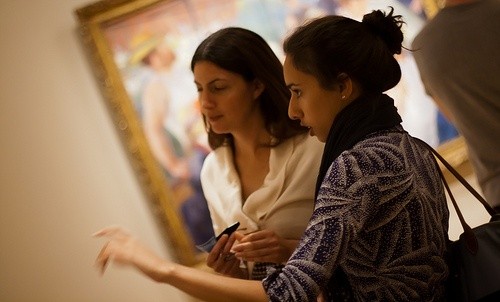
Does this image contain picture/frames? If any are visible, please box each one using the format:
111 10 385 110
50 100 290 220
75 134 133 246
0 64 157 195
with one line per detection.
77 0 469 266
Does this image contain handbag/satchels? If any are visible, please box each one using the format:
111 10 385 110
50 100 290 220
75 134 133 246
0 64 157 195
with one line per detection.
412 136 500 302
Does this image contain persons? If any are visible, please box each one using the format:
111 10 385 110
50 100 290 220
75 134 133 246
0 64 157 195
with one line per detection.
93 7 450 302
412 0 500 213
191 27 325 280
